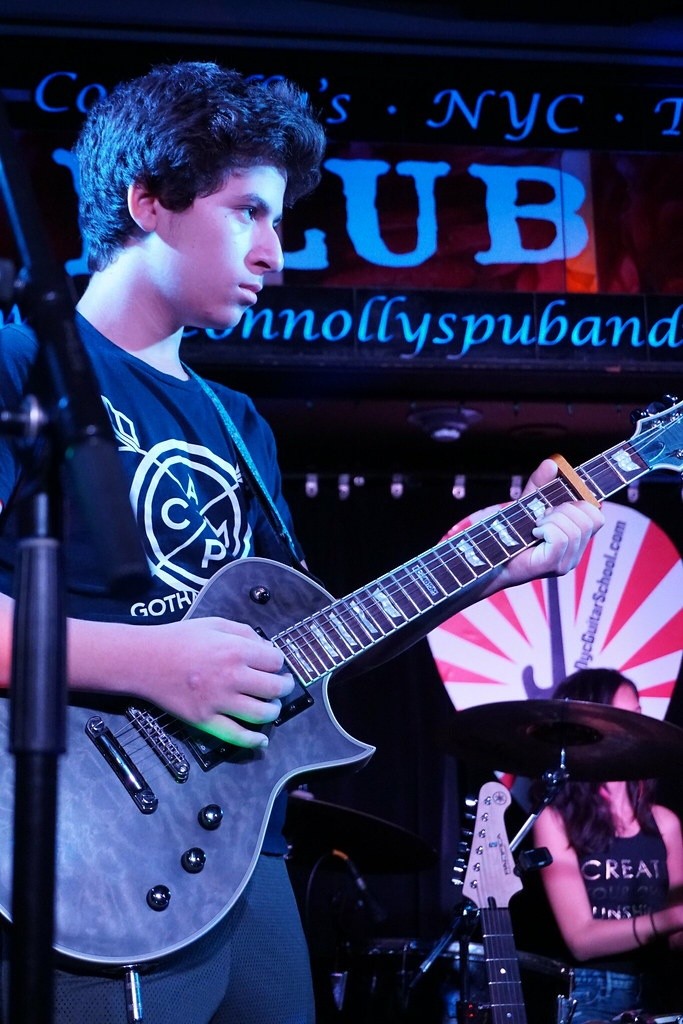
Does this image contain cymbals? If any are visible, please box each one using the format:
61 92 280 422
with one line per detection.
453 694 683 784
286 790 411 844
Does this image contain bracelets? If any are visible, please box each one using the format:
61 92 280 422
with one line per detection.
633 911 659 948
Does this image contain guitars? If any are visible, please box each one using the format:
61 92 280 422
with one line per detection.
0 388 682 983
449 780 532 1023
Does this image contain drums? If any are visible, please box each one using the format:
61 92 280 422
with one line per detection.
363 933 578 1024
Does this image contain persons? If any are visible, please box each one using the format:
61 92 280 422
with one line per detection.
534 669 683 1024
0 62 603 1024
